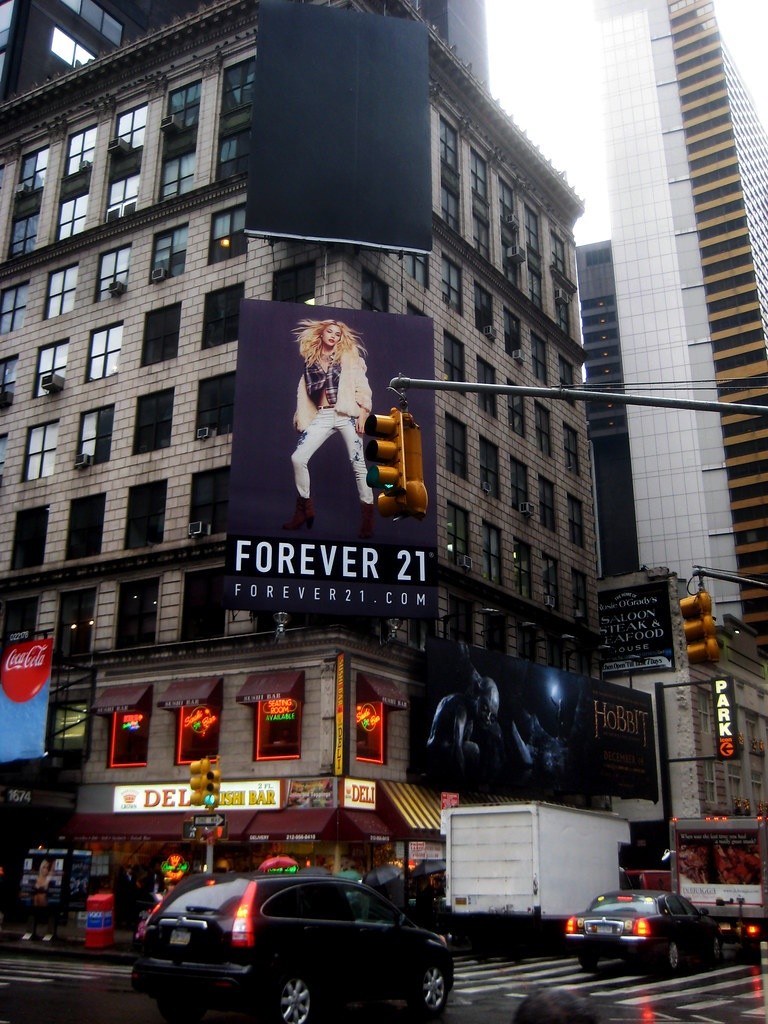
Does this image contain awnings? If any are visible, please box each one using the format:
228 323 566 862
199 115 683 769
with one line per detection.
236 671 305 710
357 674 409 713
157 678 223 717
90 684 153 720
56 810 397 846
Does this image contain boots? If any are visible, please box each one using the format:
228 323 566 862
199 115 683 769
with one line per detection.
284 496 315 529
360 502 375 538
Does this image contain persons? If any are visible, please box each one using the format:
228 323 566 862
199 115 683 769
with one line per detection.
33 859 54 906
283 319 375 538
118 865 138 930
510 989 602 1024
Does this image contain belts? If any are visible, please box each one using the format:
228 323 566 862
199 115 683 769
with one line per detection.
318 405 335 409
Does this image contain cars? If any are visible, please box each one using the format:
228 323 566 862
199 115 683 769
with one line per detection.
132 873 455 1024
564 888 724 972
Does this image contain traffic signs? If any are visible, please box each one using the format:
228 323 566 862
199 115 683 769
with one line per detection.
193 813 227 827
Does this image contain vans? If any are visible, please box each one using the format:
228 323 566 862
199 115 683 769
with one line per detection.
626 869 672 892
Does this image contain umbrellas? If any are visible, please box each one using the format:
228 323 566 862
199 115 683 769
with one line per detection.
297 867 332 875
259 855 296 871
366 864 401 887
334 870 361 880
412 860 446 876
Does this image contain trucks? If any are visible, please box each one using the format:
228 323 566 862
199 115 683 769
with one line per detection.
668 816 767 939
439 800 633 956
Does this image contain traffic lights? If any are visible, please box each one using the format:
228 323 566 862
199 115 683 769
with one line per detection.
679 591 720 664
204 769 220 808
189 759 210 805
362 407 405 519
